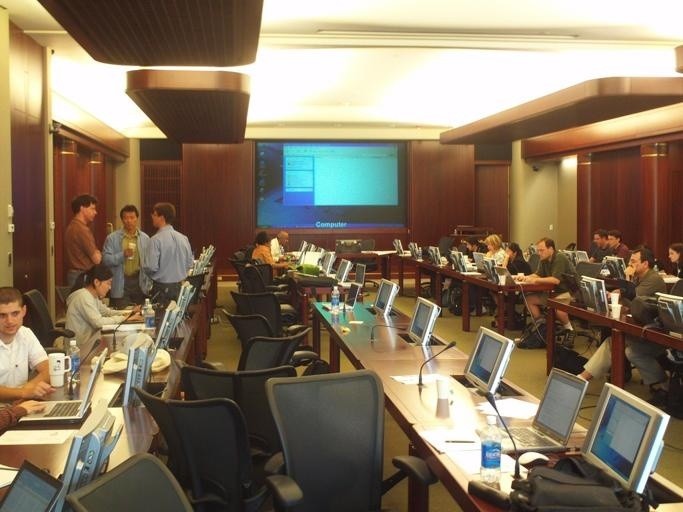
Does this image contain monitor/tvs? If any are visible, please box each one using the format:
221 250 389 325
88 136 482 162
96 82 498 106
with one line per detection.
462 325 517 395
581 380 671 494
407 295 441 345
577 250 589 264
55 397 124 512
296 239 366 287
579 275 609 313
605 255 626 279
371 279 401 317
393 236 519 286
175 279 197 318
123 332 158 407
193 242 216 276
653 290 683 331
154 300 182 351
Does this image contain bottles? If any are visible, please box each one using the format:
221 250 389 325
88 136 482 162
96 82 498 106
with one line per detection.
480 416 502 490
331 286 340 322
145 304 156 339
653 258 659 272
141 298 150 313
66 340 80 382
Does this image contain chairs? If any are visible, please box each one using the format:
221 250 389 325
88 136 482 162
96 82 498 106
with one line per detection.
234 251 245 258
670 279 683 297
183 359 327 475
254 262 274 283
264 370 439 512
230 290 306 336
135 386 285 512
575 262 605 278
64 452 193 511
528 254 539 273
57 285 75 309
436 235 456 254
201 327 312 377
25 289 74 349
228 257 250 290
222 309 318 368
234 263 297 320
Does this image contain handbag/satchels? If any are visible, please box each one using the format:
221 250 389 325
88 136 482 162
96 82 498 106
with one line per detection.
507 453 644 512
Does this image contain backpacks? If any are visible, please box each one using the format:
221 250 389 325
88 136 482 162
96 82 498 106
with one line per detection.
516 316 547 349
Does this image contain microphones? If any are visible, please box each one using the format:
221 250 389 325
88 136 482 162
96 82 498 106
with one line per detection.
112 307 137 354
69 337 100 396
342 293 370 315
483 387 525 483
368 322 408 342
419 336 459 397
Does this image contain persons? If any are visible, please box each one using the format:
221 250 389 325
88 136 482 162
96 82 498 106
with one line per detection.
450 227 683 420
104 205 152 311
146 202 196 335
1 399 45 432
271 231 295 263
0 287 56 401
252 231 289 279
244 240 258 259
60 194 103 291
66 263 141 364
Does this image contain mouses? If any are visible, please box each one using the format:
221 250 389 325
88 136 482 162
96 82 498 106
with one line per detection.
520 442 553 466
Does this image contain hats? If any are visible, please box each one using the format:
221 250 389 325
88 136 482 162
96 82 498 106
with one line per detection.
100 332 171 376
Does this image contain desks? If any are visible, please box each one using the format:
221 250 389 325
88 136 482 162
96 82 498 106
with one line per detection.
286 250 391 282
281 259 367 345
393 250 555 334
1 310 199 497
311 301 683 512
187 259 219 357
605 267 679 296
545 295 682 397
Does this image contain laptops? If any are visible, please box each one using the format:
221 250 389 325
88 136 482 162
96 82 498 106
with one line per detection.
18 344 110 427
0 458 64 512
499 365 589 453
322 282 360 312
561 271 584 304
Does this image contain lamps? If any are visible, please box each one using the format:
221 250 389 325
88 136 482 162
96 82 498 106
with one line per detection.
88 151 104 164
59 139 78 155
640 144 668 158
576 154 601 166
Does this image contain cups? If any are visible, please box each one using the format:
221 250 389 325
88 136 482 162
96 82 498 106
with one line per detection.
612 306 620 320
48 353 71 387
128 243 136 259
518 273 524 282
611 293 620 304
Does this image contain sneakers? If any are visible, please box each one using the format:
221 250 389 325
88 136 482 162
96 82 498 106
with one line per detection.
647 379 670 408
561 328 577 348
